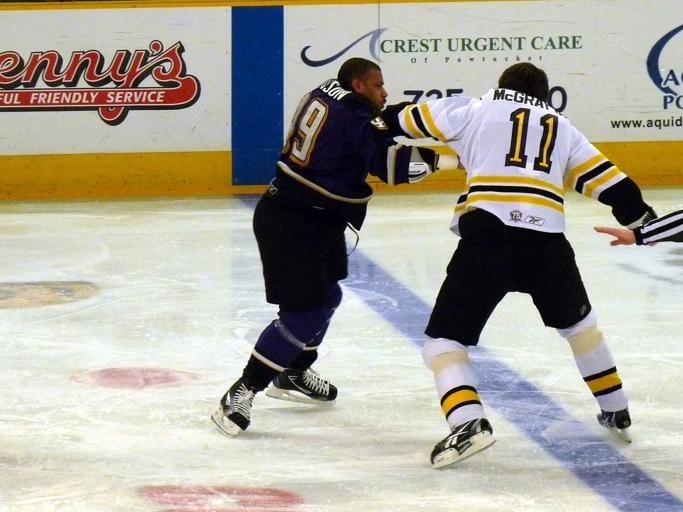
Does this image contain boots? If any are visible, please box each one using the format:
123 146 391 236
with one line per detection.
210 376 258 438
265 367 338 406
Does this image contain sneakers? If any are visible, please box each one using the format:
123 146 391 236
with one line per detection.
596 408 633 443
431 417 496 470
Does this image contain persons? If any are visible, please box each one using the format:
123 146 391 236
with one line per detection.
204 58 463 430
591 207 682 246
384 60 654 465
